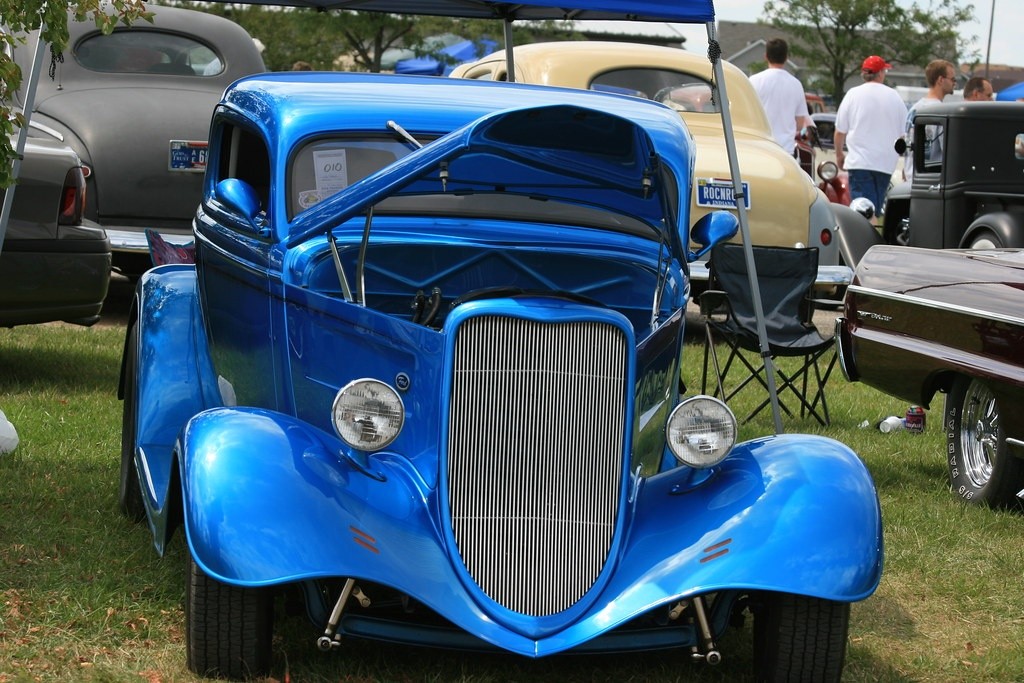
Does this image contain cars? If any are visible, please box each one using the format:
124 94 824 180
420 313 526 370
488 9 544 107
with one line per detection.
0 128 114 330
448 39 884 289
0 0 270 280
396 32 500 77
660 80 1024 252
833 246 1024 514
115 69 883 683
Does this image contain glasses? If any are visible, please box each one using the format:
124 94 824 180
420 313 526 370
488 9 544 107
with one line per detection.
883 68 888 73
943 76 956 82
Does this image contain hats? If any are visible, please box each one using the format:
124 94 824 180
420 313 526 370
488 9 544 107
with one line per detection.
863 56 891 73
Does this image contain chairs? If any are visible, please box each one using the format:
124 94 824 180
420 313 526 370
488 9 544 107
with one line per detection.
694 241 846 427
150 62 198 77
293 146 397 216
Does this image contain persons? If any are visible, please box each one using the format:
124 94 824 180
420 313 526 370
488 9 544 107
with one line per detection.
749 38 809 160
834 56 908 225
963 77 996 102
904 61 964 188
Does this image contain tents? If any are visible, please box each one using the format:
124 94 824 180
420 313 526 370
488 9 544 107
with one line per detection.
997 81 1024 100
395 38 500 76
0 0 785 439
